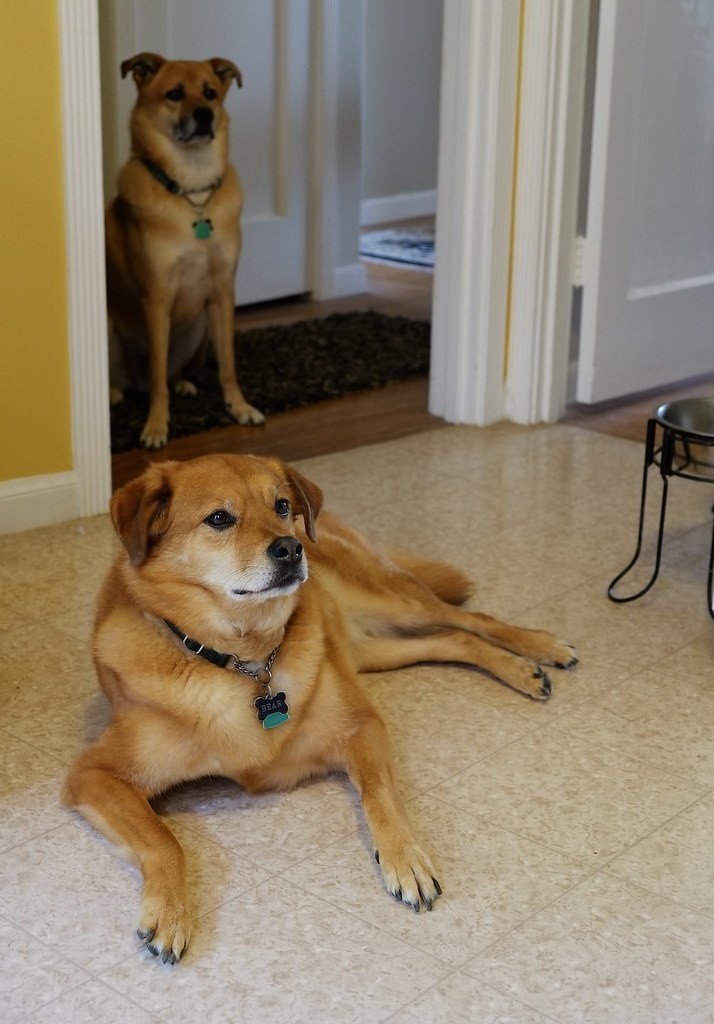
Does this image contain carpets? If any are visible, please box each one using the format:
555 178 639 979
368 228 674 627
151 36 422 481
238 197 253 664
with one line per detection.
111 309 430 451
359 221 435 272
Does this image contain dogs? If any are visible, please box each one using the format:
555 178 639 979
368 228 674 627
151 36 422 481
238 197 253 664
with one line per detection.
61 452 581 966
104 50 266 450
